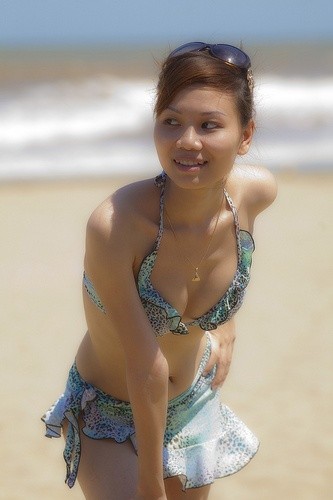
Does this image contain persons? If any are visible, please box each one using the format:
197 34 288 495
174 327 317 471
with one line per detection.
41 42 278 500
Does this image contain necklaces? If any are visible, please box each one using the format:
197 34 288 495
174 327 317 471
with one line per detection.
164 192 225 282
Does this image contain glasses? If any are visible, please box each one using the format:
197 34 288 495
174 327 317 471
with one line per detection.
168 41 252 72
